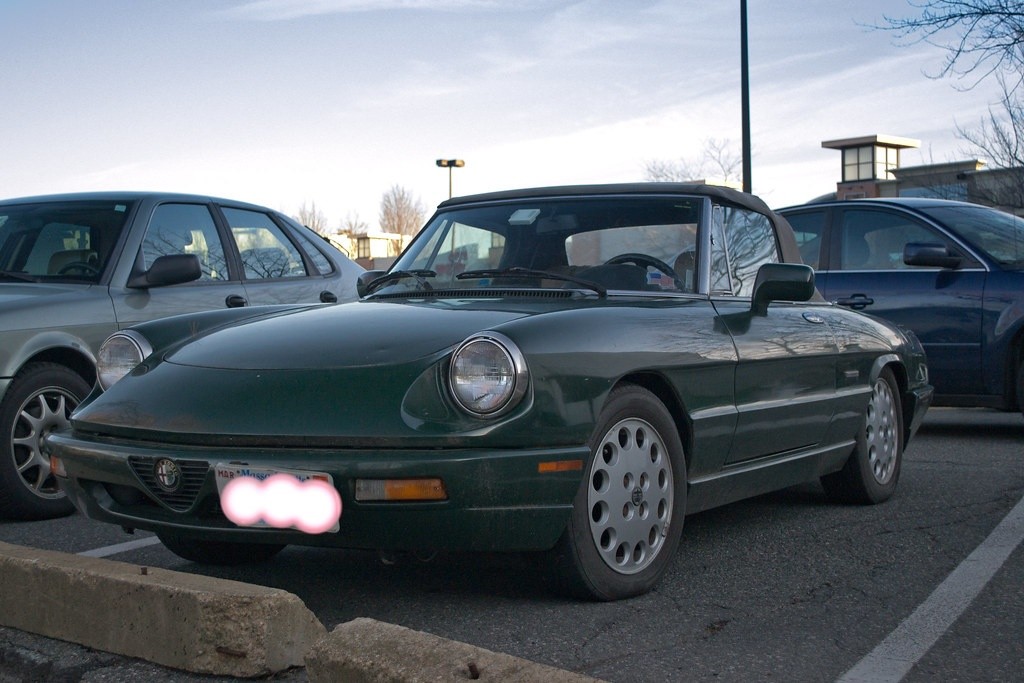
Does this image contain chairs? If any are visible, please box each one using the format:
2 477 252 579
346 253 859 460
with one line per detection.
674 251 696 293
841 237 873 270
48 250 97 277
560 265 648 290
240 248 290 278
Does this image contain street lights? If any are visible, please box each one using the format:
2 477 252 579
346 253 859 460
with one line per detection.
437 159 466 256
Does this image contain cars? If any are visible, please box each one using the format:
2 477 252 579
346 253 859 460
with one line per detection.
651 198 1024 411
0 193 367 519
37 181 932 600
